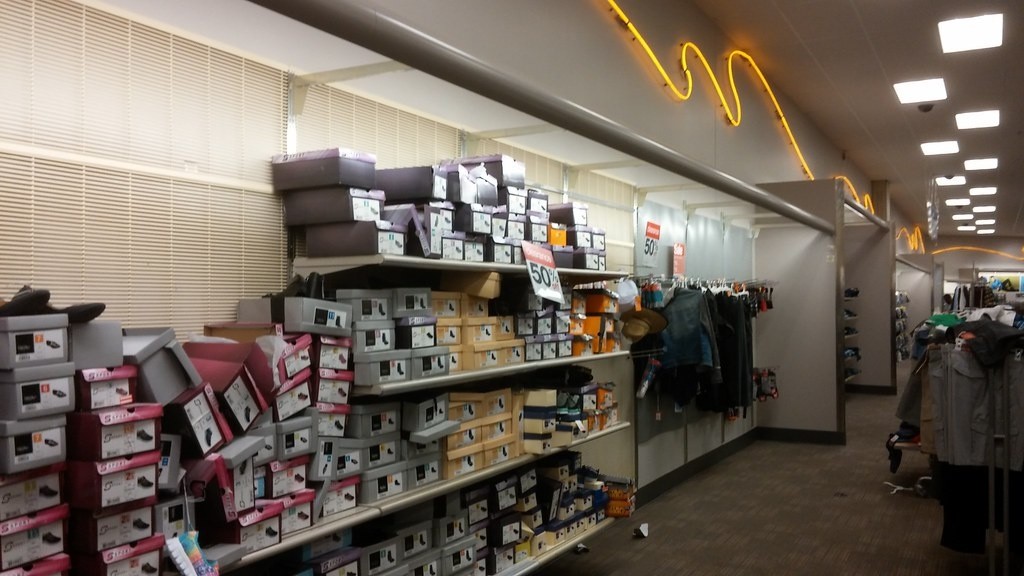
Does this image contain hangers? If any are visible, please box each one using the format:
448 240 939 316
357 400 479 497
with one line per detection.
629 274 750 299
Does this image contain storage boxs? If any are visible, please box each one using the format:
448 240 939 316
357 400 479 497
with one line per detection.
0 147 633 576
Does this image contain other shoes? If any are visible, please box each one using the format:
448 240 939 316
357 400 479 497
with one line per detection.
306 273 323 299
0 285 50 316
264 277 307 318
45 298 105 323
165 530 219 576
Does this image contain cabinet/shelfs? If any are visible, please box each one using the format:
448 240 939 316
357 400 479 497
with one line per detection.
842 294 861 382
216 254 633 575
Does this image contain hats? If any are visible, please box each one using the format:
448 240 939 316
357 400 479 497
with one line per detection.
618 308 667 342
614 277 639 320
